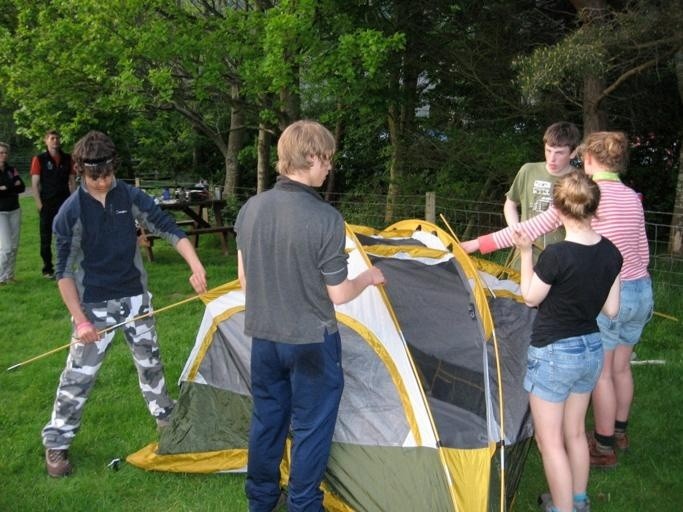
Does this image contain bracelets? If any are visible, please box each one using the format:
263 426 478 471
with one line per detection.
75 321 94 332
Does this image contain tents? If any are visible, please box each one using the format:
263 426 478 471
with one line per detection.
6 219 535 507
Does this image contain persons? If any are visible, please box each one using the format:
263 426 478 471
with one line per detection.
0 141 26 286
458 131 652 468
31 131 73 277
41 132 208 478
504 123 583 274
233 120 384 508
513 170 624 508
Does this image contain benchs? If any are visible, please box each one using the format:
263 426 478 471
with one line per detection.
135 225 241 247
131 218 202 236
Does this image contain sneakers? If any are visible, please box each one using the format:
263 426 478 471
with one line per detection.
42 271 56 279
537 490 591 512
44 446 72 478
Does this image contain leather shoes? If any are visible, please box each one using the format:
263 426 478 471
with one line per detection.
587 433 630 453
588 447 618 469
